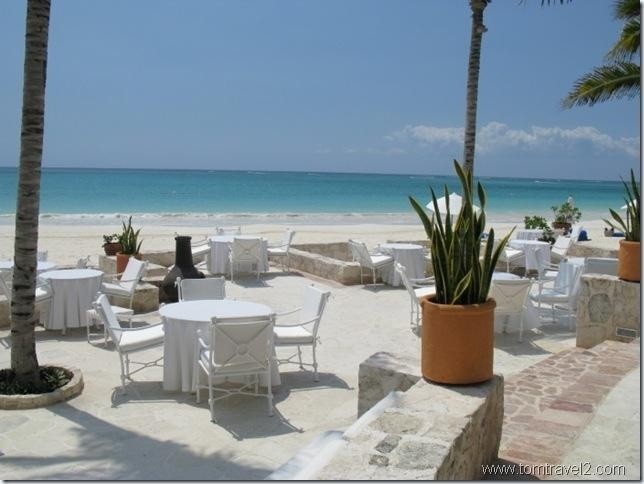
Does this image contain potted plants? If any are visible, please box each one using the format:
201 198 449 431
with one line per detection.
102 234 121 255
409 160 517 385
117 217 144 273
601 168 641 283
550 203 581 235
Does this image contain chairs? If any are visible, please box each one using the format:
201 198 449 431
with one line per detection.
0 251 164 395
157 227 331 424
348 223 585 343
